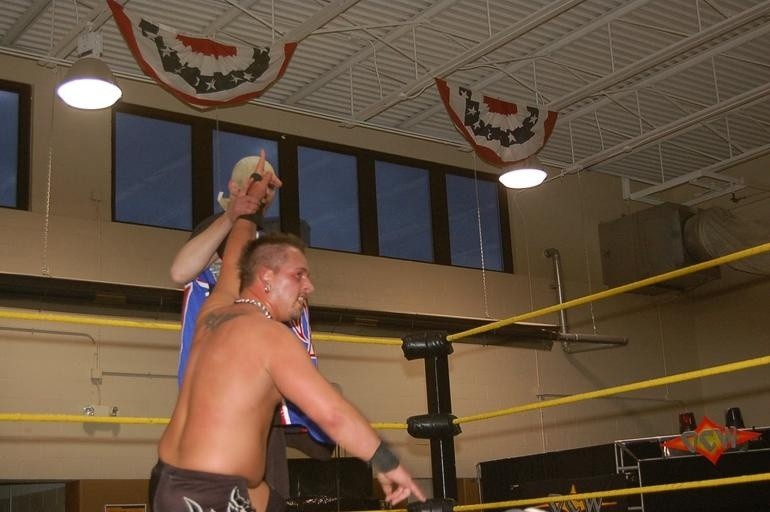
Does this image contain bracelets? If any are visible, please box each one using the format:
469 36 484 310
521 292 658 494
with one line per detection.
370 444 401 473
239 196 264 229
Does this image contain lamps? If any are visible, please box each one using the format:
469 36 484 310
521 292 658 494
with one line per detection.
55 18 123 111
499 152 547 189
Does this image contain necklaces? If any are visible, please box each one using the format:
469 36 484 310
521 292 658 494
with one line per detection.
233 296 274 321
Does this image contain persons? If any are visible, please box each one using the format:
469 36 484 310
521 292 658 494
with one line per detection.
168 156 332 512
147 148 428 511
